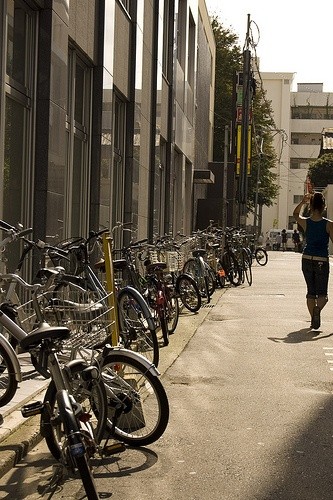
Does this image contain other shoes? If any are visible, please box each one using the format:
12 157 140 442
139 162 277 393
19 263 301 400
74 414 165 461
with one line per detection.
295 251 298 252
312 306 320 329
283 250 284 252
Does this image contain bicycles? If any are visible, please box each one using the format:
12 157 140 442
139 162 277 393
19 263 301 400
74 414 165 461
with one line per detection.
0 266 126 500
0 219 268 447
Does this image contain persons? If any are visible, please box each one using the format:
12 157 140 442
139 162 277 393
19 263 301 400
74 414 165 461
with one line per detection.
275 232 281 251
293 192 333 329
292 230 300 252
281 229 288 251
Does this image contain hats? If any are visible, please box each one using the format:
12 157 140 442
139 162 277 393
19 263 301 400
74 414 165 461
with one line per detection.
294 230 296 232
282 229 285 232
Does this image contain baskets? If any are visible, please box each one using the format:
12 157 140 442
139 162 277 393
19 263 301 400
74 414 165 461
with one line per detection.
149 251 184 272
48 236 88 276
17 290 115 349
180 236 196 248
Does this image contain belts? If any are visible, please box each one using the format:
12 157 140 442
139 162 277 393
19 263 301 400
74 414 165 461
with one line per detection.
302 255 327 261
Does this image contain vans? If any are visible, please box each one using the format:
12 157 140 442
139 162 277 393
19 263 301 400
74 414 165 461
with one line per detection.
269 229 303 251
